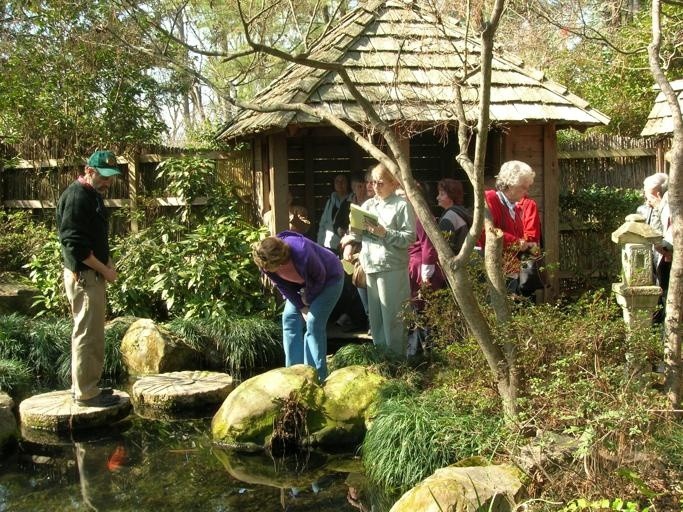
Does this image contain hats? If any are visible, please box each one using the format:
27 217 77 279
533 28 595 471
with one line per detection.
86 150 124 178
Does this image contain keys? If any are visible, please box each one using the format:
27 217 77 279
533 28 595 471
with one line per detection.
77 271 86 288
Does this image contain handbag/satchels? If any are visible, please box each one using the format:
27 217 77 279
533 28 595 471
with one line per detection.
350 257 367 289
519 251 553 298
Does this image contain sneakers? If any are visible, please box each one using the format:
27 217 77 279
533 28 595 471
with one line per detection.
334 313 353 328
72 387 114 404
75 392 121 407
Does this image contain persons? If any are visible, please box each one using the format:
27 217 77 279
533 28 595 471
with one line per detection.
56 151 123 406
634 173 674 330
